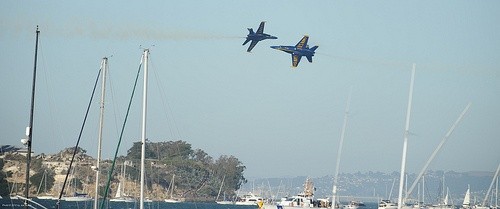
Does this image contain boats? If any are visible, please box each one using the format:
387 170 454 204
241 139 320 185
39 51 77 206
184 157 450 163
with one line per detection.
212 64 500 209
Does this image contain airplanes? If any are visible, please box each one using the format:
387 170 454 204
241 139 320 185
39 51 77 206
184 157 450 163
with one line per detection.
242 20 279 53
269 33 319 68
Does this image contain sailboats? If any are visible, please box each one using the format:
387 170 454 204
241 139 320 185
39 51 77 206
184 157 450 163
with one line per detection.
164 172 182 204
